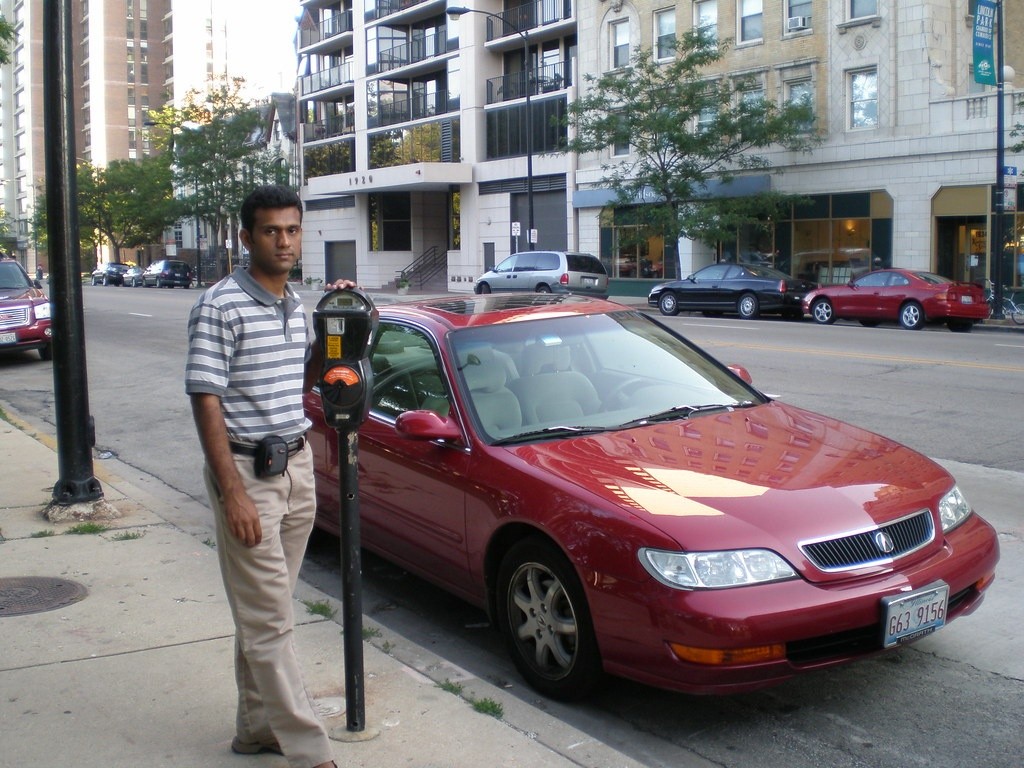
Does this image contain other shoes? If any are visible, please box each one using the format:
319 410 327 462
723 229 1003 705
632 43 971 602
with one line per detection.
231 736 283 754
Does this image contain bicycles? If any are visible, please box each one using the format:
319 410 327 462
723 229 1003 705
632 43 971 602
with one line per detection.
984 278 1024 325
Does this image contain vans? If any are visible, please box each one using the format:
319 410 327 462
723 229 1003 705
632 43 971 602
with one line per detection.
474 250 610 301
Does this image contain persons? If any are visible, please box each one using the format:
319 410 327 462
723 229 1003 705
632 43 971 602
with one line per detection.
186 183 357 768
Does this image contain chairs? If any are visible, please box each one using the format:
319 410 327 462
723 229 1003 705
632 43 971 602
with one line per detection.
421 346 521 431
507 341 606 425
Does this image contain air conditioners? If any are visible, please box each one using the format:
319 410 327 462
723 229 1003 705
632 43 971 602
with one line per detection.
787 15 808 30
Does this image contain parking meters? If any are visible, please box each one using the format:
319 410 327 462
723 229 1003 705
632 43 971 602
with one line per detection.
313 286 381 731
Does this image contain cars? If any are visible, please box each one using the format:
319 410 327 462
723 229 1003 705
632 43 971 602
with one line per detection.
121 266 146 288
802 268 991 333
91 263 131 286
0 259 53 361
142 260 192 289
303 293 999 705
647 263 817 322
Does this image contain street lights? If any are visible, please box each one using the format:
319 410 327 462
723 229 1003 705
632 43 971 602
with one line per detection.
446 7 535 251
144 122 202 288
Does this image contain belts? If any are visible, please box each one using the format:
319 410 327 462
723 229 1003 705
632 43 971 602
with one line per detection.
229 432 307 455
